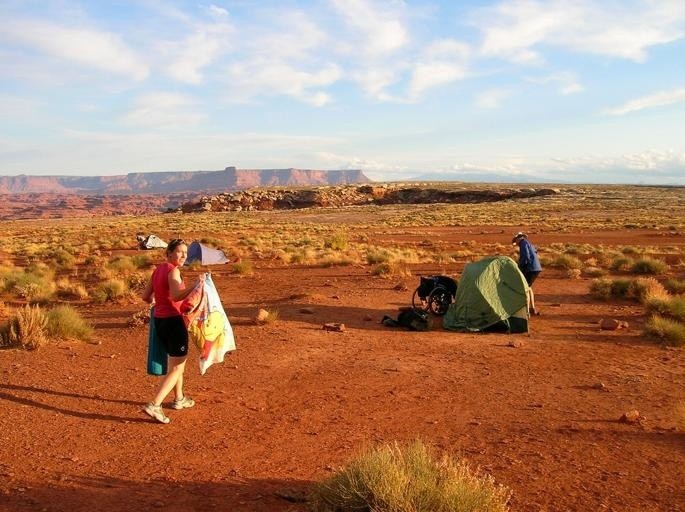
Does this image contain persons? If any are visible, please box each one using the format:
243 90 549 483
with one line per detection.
512 232 542 320
142 239 206 424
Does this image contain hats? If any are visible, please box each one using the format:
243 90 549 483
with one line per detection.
512 232 528 244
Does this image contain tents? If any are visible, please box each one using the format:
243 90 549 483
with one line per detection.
185 242 230 266
142 234 168 249
443 256 530 332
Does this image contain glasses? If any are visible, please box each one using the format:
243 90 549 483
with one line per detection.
169 238 186 246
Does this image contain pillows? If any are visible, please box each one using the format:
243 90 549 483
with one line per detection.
173 273 238 376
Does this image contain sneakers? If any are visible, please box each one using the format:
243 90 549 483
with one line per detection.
171 395 195 410
143 401 169 423
529 307 536 314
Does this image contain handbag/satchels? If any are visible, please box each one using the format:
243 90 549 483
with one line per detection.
384 307 433 331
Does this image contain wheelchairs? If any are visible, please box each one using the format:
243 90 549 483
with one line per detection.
412 271 452 315
